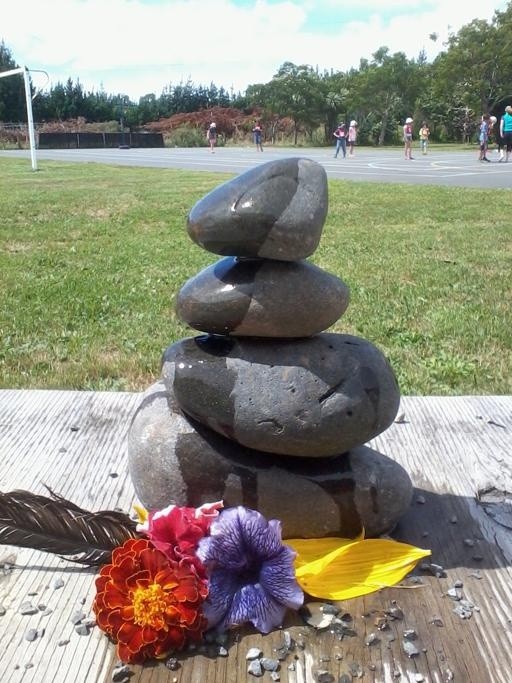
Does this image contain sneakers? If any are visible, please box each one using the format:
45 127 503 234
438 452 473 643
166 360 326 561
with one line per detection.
479 154 505 163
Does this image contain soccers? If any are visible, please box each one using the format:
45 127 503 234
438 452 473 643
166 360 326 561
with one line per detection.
490 116 497 124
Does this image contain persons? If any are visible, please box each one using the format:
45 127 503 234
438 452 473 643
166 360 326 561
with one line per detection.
483 114 497 163
251 119 264 151
418 121 430 155
332 121 349 159
479 113 488 160
348 119 358 156
402 116 414 159
497 105 511 162
206 120 218 152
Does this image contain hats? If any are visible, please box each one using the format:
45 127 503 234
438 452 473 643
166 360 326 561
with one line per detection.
337 120 358 128
404 118 414 123
421 120 428 128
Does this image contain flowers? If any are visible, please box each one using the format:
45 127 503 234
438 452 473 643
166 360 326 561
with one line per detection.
91 498 432 663
